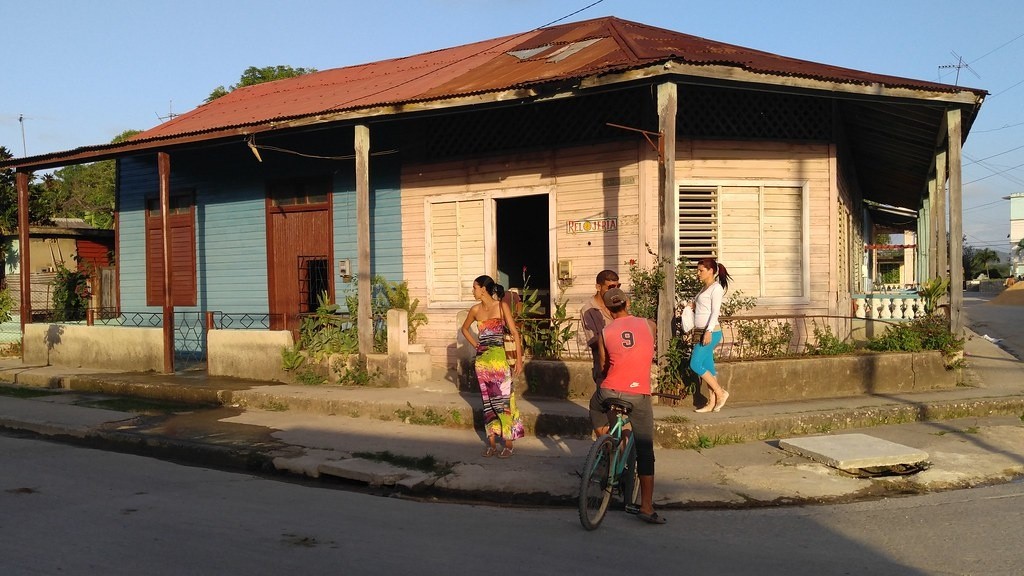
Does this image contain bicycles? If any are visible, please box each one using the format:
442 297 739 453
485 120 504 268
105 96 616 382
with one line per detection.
577 392 682 531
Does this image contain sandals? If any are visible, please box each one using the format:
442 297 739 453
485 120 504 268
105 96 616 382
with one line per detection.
482 447 499 458
498 448 516 458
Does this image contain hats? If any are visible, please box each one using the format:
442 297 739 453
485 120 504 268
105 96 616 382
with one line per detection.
603 289 627 307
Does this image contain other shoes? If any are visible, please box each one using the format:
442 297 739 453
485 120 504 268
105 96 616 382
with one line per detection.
694 391 730 414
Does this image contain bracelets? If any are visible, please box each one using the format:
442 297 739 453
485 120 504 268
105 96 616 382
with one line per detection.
706 330 712 333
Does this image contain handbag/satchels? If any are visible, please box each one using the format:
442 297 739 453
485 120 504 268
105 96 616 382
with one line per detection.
682 305 696 333
503 334 525 368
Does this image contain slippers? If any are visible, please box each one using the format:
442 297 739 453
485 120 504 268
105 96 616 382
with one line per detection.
637 512 667 524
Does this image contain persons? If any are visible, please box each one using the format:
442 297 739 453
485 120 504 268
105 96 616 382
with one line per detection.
686 258 729 413
581 269 631 366
461 275 525 458
589 288 667 524
1006 275 1014 287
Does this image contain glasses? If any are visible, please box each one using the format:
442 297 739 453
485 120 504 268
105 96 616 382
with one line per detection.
603 282 622 290
